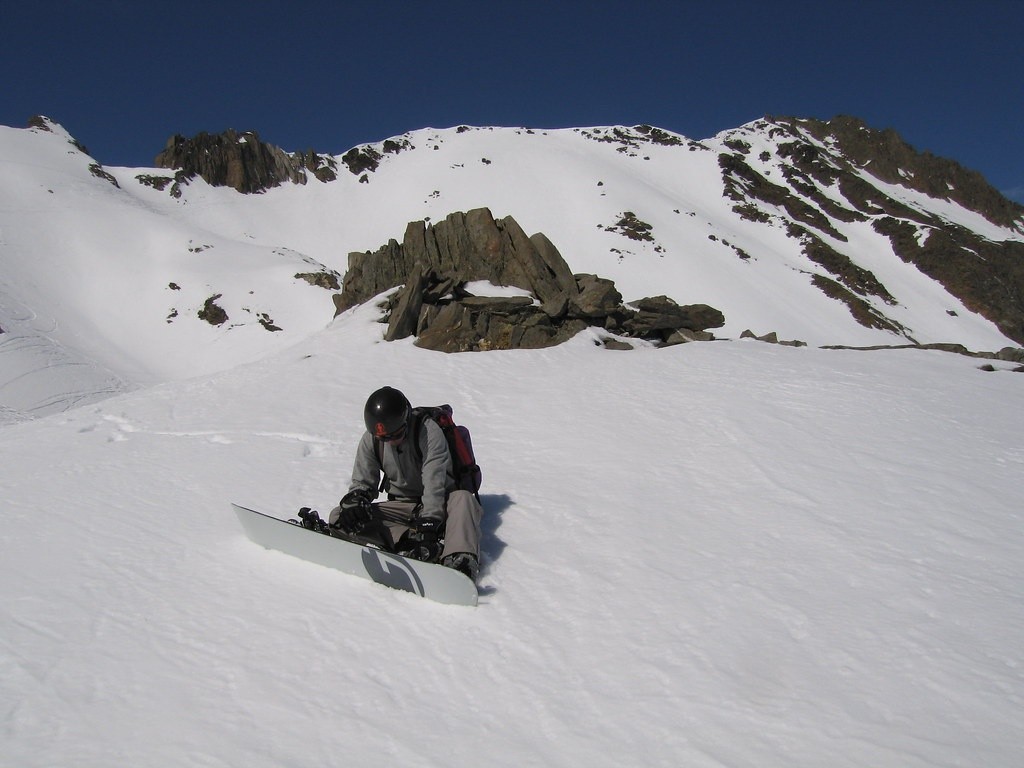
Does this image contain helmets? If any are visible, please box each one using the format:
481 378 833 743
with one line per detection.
363 386 412 437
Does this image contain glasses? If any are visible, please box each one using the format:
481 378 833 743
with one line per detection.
376 426 409 442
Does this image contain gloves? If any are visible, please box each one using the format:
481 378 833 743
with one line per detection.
339 489 373 537
408 517 445 562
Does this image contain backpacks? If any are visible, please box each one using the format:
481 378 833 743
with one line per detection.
411 404 482 506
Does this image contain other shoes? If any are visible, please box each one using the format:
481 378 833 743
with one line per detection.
437 552 480 584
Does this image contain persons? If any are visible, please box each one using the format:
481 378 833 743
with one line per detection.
328 386 483 580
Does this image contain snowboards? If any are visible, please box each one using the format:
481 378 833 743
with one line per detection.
229 502 479 608
